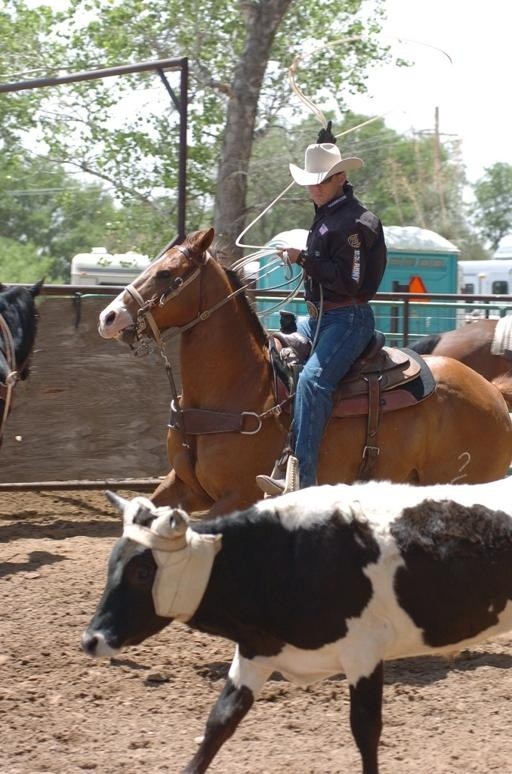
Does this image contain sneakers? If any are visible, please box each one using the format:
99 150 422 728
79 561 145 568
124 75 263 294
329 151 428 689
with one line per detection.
256 474 318 497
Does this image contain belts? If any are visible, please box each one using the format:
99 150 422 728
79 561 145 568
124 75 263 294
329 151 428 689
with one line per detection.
308 293 368 313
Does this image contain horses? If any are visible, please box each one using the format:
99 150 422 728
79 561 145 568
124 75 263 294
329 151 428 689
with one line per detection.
0 274 47 448
404 315 512 378
91 222 512 516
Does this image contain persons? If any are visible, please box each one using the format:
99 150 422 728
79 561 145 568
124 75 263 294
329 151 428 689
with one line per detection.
256 143 388 497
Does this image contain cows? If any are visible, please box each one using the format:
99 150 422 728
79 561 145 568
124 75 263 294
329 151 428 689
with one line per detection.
78 466 512 774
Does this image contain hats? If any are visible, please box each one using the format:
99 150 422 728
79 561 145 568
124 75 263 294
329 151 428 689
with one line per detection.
288 143 365 186
